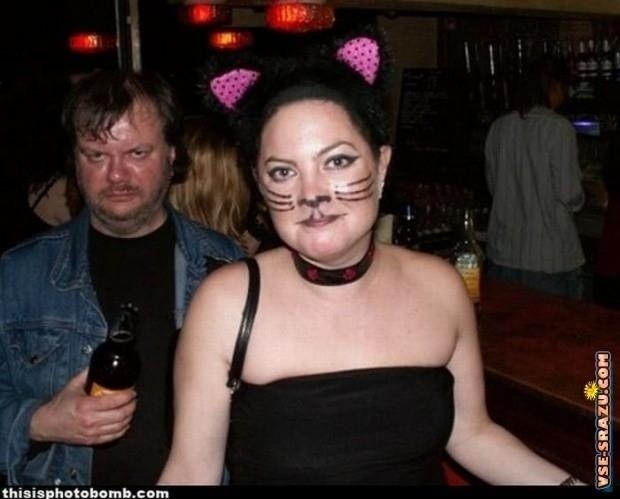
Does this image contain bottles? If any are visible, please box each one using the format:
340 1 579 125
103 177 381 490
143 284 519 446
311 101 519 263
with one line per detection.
399 204 420 252
540 34 620 99
81 301 145 401
453 206 486 329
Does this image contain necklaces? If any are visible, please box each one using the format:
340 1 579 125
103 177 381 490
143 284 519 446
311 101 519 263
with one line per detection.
288 231 376 285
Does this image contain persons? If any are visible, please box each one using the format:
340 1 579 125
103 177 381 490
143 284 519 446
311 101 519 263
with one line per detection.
0 144 84 253
238 176 282 256
479 54 598 302
156 37 594 487
592 134 618 309
1 64 247 487
169 111 253 241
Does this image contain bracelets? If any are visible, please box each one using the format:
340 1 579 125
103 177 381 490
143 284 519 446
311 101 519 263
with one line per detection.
559 472 580 485
